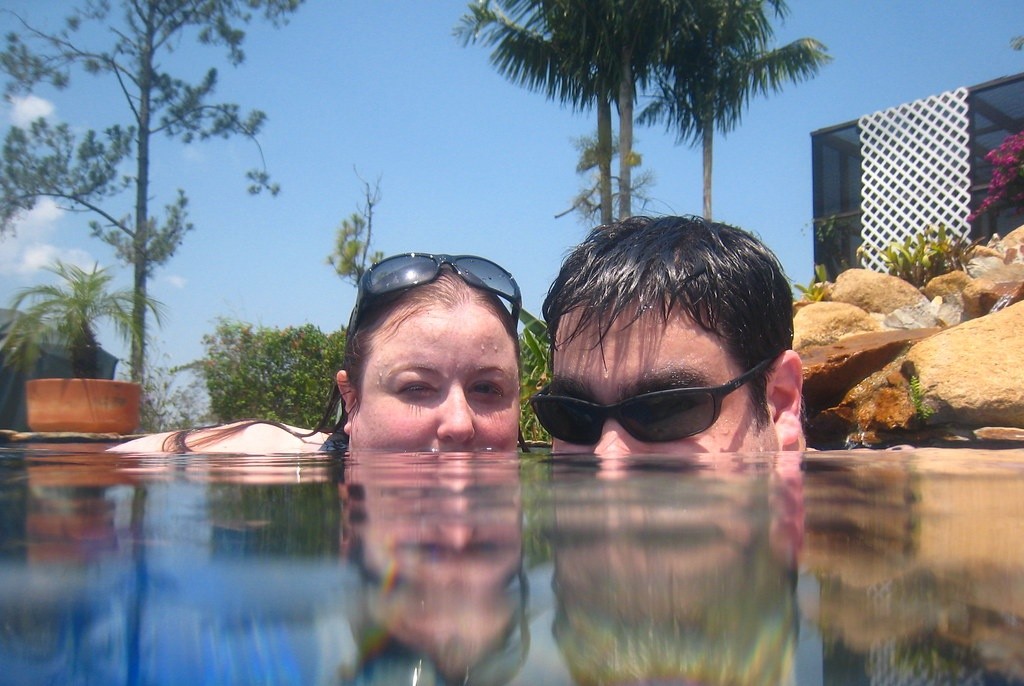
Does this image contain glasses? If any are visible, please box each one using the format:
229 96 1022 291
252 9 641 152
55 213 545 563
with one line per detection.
529 356 777 445
346 254 523 345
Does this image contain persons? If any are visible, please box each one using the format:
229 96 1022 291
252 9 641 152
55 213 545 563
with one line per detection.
531 216 818 454
103 252 520 453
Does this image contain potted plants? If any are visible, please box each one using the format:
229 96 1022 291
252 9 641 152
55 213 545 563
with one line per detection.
8 464 173 683
0 257 168 431
